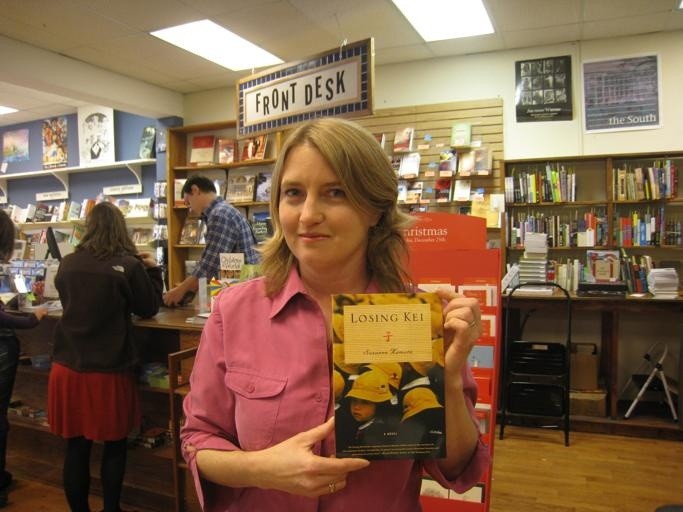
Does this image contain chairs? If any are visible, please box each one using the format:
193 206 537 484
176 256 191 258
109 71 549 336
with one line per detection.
615 340 679 423
498 282 573 446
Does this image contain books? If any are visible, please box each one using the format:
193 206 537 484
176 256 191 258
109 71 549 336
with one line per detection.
503 164 576 204
371 133 385 157
18 349 45 367
437 147 492 177
245 211 275 245
621 245 650 294
612 205 682 245
8 398 47 427
645 266 678 297
393 129 414 153
330 292 447 456
613 158 678 201
132 420 167 450
500 232 581 294
397 177 483 205
189 136 216 162
134 358 169 390
173 175 275 204
472 195 504 228
388 154 420 178
217 137 238 163
450 124 471 147
505 208 607 249
242 133 267 161
5 200 153 221
177 212 208 245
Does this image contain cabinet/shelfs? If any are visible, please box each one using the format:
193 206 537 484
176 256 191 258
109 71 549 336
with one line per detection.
0 158 167 305
504 153 683 437
167 117 290 305
0 305 215 512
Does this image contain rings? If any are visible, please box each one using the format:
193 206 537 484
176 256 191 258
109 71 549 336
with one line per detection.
329 483 335 493
469 322 477 327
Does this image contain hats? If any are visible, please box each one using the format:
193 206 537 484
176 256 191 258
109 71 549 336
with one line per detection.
361 362 403 390
430 339 446 369
409 352 435 378
333 344 361 374
333 293 444 343
344 372 394 402
400 387 444 422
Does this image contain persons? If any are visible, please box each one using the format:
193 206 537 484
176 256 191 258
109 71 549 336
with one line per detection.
179 117 489 512
161 176 256 306
47 202 163 512
0 208 48 507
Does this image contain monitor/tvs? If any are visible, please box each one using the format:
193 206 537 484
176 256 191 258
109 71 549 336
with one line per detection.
45 227 62 261
